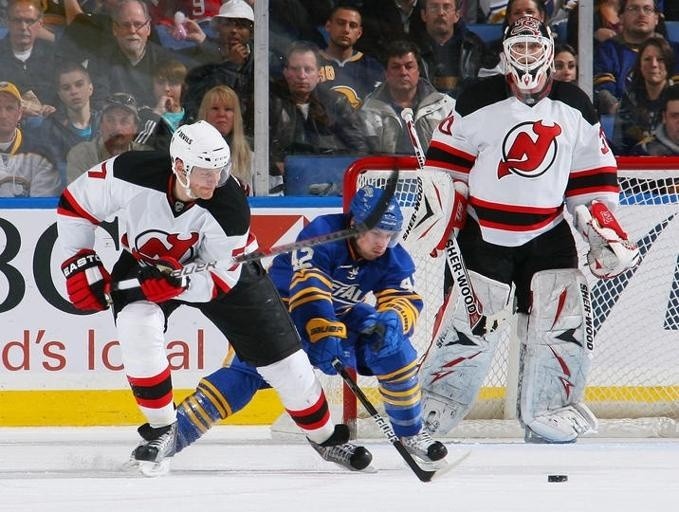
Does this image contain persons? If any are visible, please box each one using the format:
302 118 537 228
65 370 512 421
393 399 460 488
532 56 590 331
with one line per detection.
135 184 448 463
0 0 679 198
401 16 640 444
56 120 372 471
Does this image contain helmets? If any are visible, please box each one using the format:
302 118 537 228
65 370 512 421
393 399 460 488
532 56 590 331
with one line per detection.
502 14 554 91
350 184 403 231
169 119 230 189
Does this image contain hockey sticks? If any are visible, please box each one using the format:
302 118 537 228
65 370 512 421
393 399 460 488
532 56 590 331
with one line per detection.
401 106 526 336
103 167 400 298
332 360 483 484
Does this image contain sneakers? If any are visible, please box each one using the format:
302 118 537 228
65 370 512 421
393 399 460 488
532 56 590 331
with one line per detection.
135 423 178 462
401 432 448 462
307 425 372 470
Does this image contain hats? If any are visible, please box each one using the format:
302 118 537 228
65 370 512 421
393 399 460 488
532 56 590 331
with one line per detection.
213 0 254 21
0 81 21 100
103 91 139 117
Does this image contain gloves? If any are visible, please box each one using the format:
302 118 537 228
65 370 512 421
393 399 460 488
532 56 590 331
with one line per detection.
61 249 111 311
360 310 401 360
310 337 347 375
138 256 189 303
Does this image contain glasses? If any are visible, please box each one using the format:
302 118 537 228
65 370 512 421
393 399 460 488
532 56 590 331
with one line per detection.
104 95 135 105
